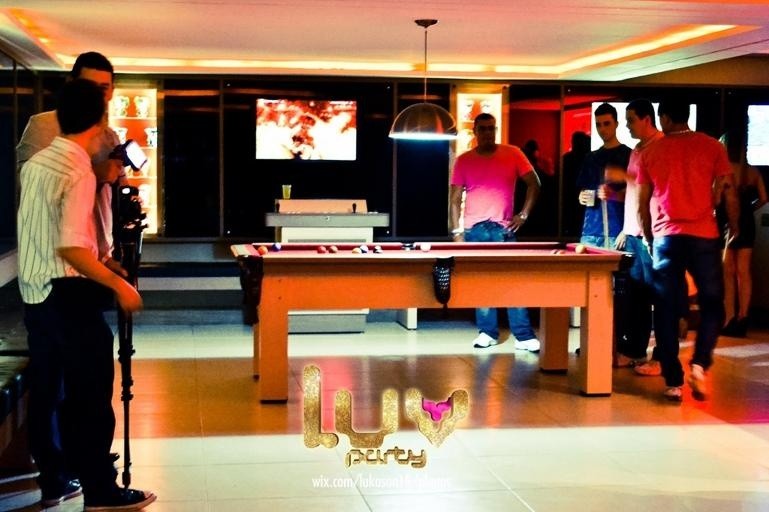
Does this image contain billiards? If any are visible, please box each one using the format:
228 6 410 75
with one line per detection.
352 245 368 253
329 246 337 253
373 246 381 253
317 246 326 253
272 243 281 251
257 246 268 255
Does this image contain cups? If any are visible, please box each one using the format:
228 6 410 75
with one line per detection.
585 190 596 207
282 184 292 199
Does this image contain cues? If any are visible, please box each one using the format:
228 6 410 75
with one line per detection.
601 199 609 247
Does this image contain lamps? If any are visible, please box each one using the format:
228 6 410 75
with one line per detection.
384 17 463 141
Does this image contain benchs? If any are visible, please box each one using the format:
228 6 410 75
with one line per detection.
274 197 370 215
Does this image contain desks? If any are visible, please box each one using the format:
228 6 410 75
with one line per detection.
225 236 632 406
265 209 392 244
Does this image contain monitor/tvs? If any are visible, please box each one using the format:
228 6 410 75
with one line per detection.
253 95 359 165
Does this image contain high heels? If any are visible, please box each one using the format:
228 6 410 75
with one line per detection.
721 315 748 337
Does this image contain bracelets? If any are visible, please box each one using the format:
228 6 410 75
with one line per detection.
450 227 461 238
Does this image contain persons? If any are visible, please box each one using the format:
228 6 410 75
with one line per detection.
288 136 312 160
16 78 158 511
14 52 123 472
450 113 544 352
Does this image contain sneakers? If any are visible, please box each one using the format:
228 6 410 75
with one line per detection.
473 331 499 347
636 360 664 375
664 385 682 401
108 450 119 463
613 351 648 368
514 337 541 353
85 480 156 511
41 480 84 506
